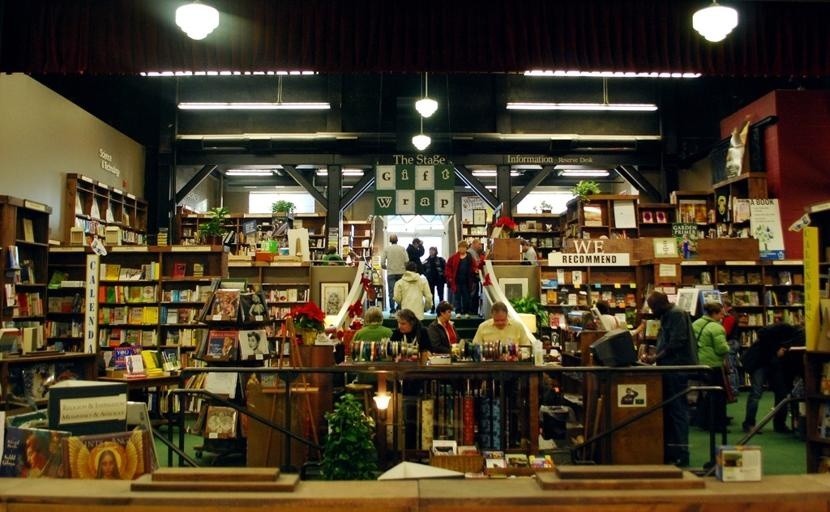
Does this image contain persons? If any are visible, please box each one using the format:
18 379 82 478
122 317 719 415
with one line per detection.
722 309 751 404
640 289 699 468
350 234 541 449
691 302 734 437
738 321 805 434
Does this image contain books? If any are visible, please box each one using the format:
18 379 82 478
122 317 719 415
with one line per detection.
532 461 706 492
541 268 803 388
553 460 685 479
130 467 302 492
151 466 280 483
1 178 371 478
463 199 785 263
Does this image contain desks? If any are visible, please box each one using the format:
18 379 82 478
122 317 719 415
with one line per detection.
238 362 711 474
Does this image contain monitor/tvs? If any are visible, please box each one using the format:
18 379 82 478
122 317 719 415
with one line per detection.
588 329 639 367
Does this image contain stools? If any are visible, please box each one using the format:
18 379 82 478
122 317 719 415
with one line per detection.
344 382 374 418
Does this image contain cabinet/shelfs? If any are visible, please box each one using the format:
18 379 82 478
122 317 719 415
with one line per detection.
475 87 830 474
1 167 384 434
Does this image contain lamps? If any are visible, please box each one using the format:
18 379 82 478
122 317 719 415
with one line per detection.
411 115 432 151
523 0 706 81
372 391 393 410
140 0 360 144
691 0 739 43
415 71 438 118
